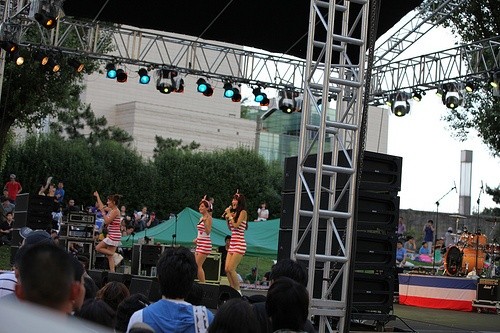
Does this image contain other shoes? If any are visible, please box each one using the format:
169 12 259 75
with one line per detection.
237 290 242 297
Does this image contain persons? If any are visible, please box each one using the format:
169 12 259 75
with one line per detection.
94 191 124 272
257 200 269 221
0 174 81 245
0 230 315 333
93 199 159 263
193 199 213 284
218 235 231 277
222 193 248 297
245 265 276 287
253 217 258 222
396 217 486 268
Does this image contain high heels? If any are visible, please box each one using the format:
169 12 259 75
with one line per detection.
112 252 124 265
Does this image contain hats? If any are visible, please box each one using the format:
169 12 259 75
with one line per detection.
10 174 16 179
19 227 54 243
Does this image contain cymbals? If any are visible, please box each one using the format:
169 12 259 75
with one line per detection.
449 215 467 219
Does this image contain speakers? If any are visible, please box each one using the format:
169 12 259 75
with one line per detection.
277 149 403 314
10 193 53 264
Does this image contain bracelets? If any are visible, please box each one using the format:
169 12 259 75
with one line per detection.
101 212 107 217
203 224 208 228
228 217 233 221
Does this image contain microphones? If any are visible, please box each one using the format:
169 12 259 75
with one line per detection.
221 205 232 217
197 220 204 225
97 205 107 212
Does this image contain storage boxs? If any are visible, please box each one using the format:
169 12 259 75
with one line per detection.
194 253 222 284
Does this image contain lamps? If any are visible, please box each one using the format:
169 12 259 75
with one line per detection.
0 9 500 118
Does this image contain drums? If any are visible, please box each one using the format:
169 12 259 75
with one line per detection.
443 245 484 277
458 232 473 243
471 234 488 247
484 243 500 252
